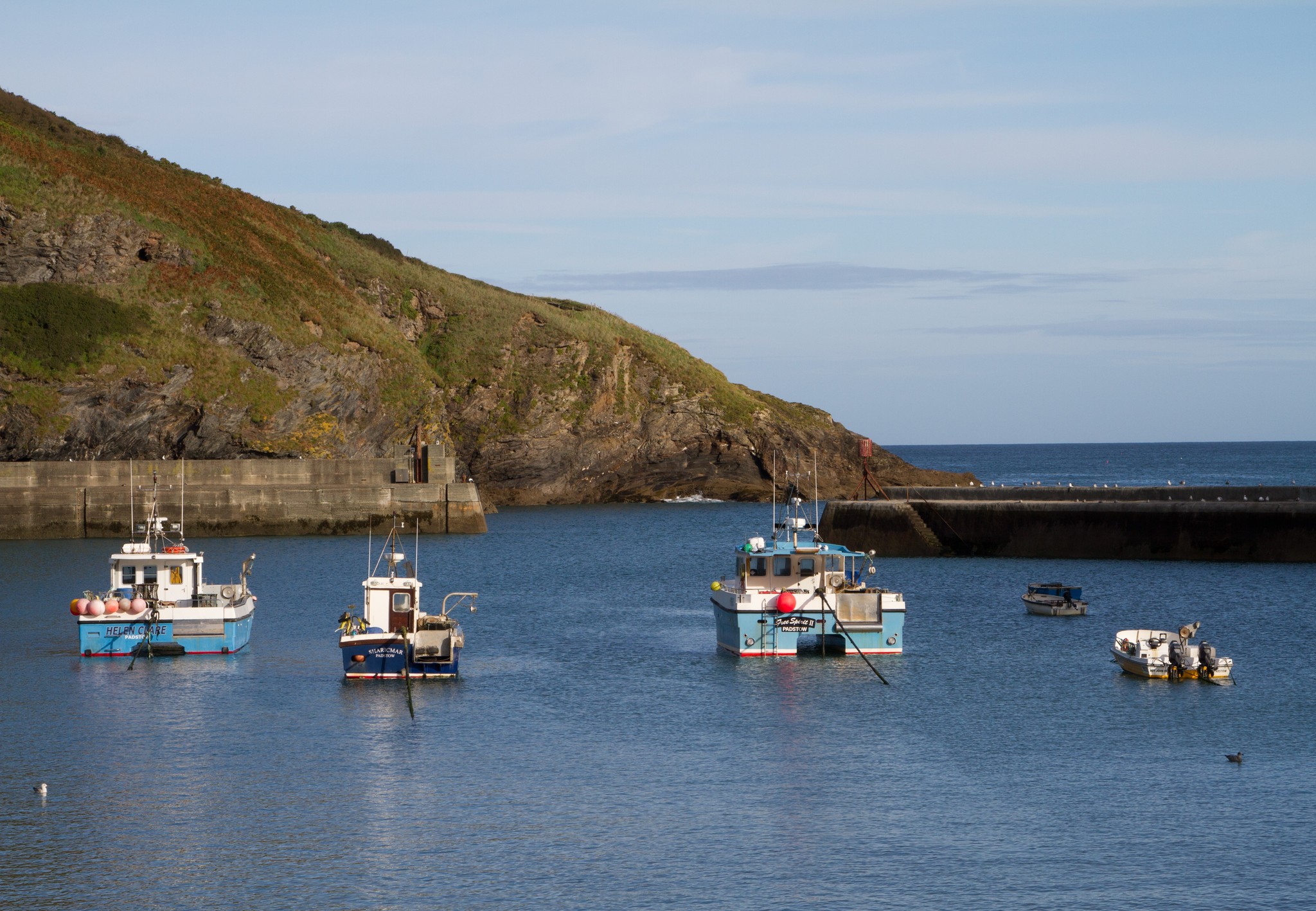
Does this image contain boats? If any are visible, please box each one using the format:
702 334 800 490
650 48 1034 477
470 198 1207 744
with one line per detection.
707 442 904 688
1021 581 1088 616
71 459 258 658
1111 621 1235 686
335 515 479 680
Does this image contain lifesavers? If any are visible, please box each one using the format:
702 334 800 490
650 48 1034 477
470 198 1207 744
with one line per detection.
162 546 185 553
1123 638 1129 650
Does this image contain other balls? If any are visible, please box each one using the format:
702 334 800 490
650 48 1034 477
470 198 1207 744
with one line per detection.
88 599 105 616
105 599 119 613
76 598 90 614
711 581 720 591
131 598 146 613
70 599 80 615
119 598 131 611
776 592 796 612
743 544 752 552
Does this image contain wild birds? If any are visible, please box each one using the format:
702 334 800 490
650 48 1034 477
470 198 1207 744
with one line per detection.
1225 480 1230 486
1295 497 1300 502
1258 483 1264 486
1291 479 1296 486
33 783 49 793
1225 752 1244 762
954 480 1223 503
1265 497 1269 502
1243 495 1248 502
1259 497 1264 502
